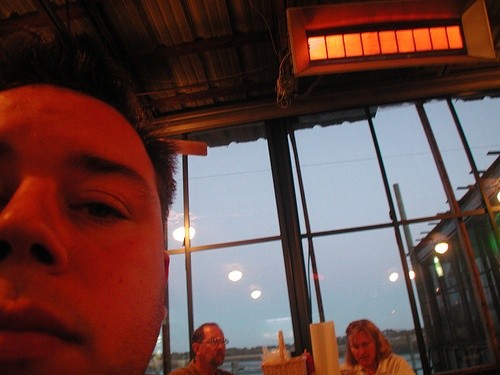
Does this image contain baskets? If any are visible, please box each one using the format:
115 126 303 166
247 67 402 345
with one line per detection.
262 330 307 375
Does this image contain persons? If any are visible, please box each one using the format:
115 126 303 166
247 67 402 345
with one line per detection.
0 42 170 375
168 322 236 375
339 319 416 375
